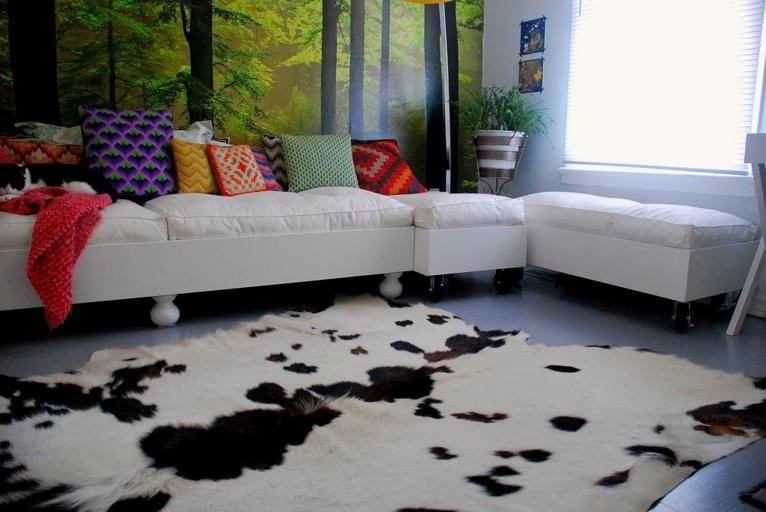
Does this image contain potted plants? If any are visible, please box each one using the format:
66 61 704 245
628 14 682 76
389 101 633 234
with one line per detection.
443 78 556 176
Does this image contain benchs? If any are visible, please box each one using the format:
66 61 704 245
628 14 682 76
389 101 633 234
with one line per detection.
513 190 758 334
0 185 416 328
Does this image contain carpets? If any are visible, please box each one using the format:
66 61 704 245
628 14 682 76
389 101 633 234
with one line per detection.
1 291 764 511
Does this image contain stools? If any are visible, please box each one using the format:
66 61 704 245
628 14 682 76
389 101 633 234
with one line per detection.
389 191 527 302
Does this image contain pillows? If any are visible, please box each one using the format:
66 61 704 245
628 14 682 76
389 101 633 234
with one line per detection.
351 138 428 196
76 105 178 202
170 120 288 196
283 135 359 192
0 123 85 197
